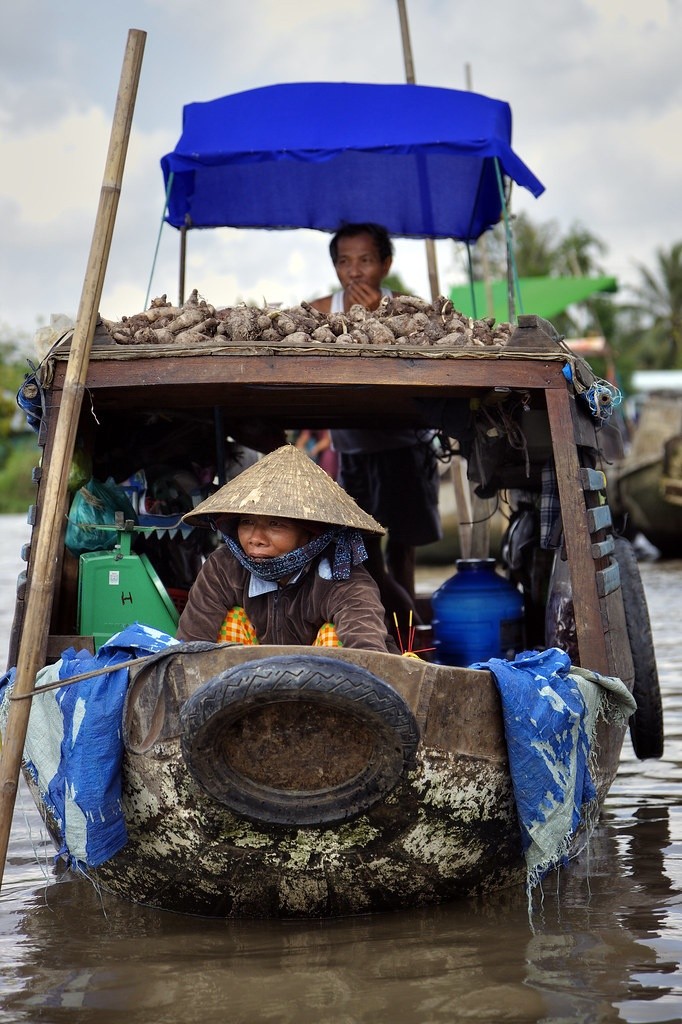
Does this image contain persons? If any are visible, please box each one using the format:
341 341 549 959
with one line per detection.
171 428 341 507
175 446 403 655
309 221 441 606
628 525 659 563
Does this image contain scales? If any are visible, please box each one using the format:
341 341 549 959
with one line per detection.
64 510 182 655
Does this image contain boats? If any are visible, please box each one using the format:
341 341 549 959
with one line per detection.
0 316 632 918
616 386 682 561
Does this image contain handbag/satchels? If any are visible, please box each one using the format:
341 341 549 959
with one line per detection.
63 471 142 555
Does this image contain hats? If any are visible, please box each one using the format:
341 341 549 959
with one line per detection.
181 442 387 538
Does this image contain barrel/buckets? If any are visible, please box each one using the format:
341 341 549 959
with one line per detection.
430 558 526 672
